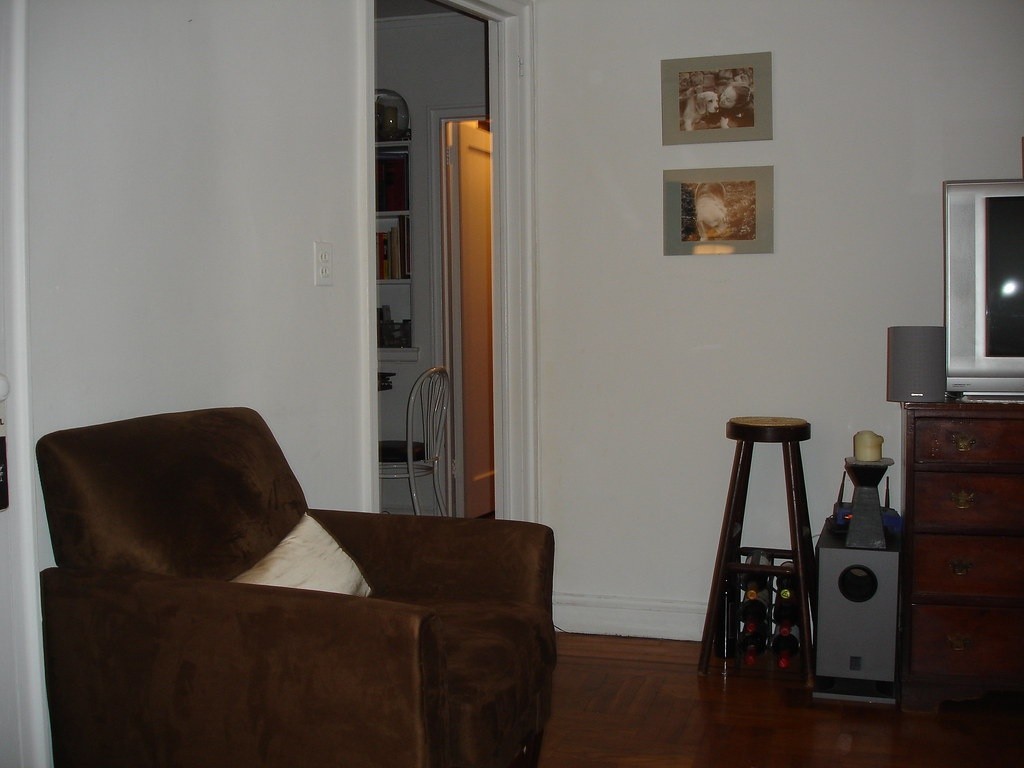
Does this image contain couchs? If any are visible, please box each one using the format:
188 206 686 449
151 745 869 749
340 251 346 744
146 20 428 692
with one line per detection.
39 406 558 768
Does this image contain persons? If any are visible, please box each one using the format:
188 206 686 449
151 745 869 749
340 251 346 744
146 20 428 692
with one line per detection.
705 80 754 127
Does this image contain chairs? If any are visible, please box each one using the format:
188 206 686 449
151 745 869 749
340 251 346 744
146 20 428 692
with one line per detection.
378 367 449 516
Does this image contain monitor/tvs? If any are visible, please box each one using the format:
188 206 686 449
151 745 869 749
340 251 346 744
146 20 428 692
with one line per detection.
943 178 1024 396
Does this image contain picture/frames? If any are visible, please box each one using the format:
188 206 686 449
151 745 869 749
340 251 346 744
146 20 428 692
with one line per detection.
663 166 774 256
660 51 774 146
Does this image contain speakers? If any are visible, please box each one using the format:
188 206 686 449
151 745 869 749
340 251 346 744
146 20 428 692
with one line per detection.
885 325 948 403
814 507 905 703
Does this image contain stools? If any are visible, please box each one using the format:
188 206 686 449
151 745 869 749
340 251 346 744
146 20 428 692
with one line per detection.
698 417 814 687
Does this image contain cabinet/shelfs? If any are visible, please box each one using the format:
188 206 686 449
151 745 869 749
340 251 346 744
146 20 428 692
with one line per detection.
374 140 419 362
900 401 1024 712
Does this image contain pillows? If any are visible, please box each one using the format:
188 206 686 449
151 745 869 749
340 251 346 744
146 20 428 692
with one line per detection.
230 513 372 599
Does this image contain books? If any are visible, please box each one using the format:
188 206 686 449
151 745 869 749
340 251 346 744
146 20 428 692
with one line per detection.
377 215 410 280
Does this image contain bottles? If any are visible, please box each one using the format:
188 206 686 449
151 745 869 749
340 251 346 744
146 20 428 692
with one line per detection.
737 548 798 670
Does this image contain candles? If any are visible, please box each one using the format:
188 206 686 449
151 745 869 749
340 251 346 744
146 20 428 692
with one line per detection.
853 430 884 461
